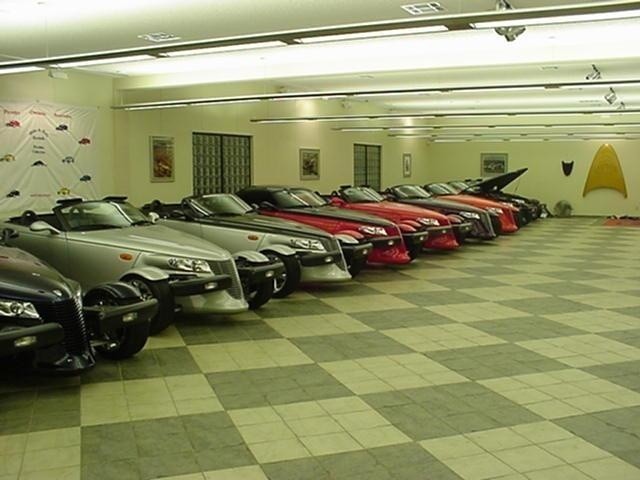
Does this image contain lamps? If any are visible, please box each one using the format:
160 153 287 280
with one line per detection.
604 88 617 105
494 1 525 42
585 63 602 85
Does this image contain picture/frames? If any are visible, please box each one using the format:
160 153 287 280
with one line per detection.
480 153 508 177
299 148 321 181
403 153 412 178
149 135 177 182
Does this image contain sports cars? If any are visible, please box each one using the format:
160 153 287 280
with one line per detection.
0 192 251 358
0 241 97 374
